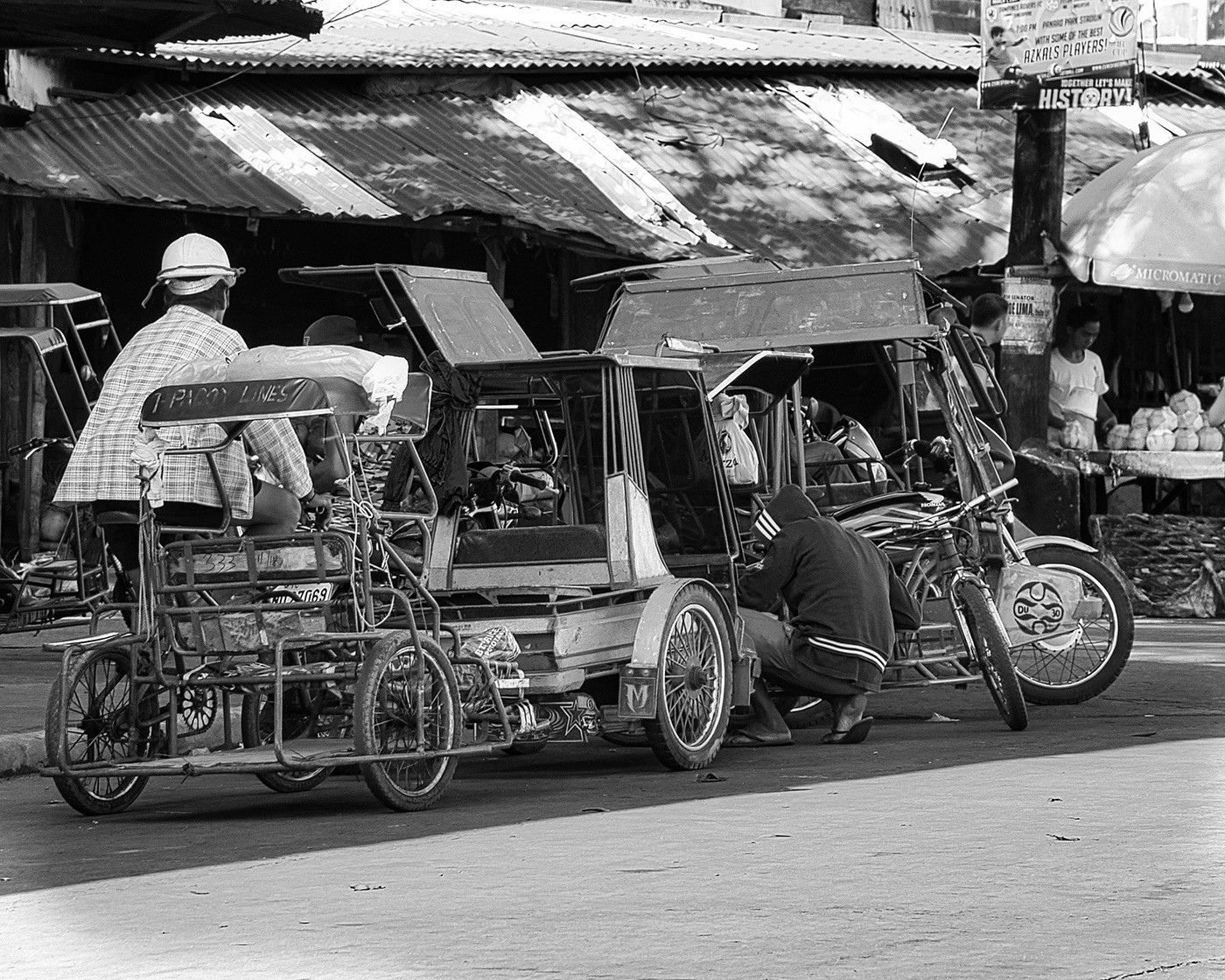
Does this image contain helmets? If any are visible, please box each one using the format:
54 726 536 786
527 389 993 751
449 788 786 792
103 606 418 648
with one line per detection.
156 232 247 287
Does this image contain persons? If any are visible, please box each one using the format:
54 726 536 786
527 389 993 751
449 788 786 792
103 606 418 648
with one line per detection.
720 483 921 747
51 232 347 707
927 293 1172 544
983 26 1058 84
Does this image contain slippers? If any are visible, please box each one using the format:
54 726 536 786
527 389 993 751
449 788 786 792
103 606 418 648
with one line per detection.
819 717 873 744
721 727 796 748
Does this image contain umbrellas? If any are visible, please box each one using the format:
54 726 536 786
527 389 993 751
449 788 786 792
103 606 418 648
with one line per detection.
1041 129 1225 296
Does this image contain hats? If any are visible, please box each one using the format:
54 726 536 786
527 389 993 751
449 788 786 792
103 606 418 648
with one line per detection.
302 316 362 348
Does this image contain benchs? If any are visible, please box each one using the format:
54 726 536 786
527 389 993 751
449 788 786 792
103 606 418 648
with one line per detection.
139 529 358 645
459 523 606 566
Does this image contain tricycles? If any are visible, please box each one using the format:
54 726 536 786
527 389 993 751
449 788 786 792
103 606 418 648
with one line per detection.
0 284 140 633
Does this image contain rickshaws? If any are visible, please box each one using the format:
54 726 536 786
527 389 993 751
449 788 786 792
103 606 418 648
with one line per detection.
36 349 761 818
570 254 1137 706
273 263 1029 732
